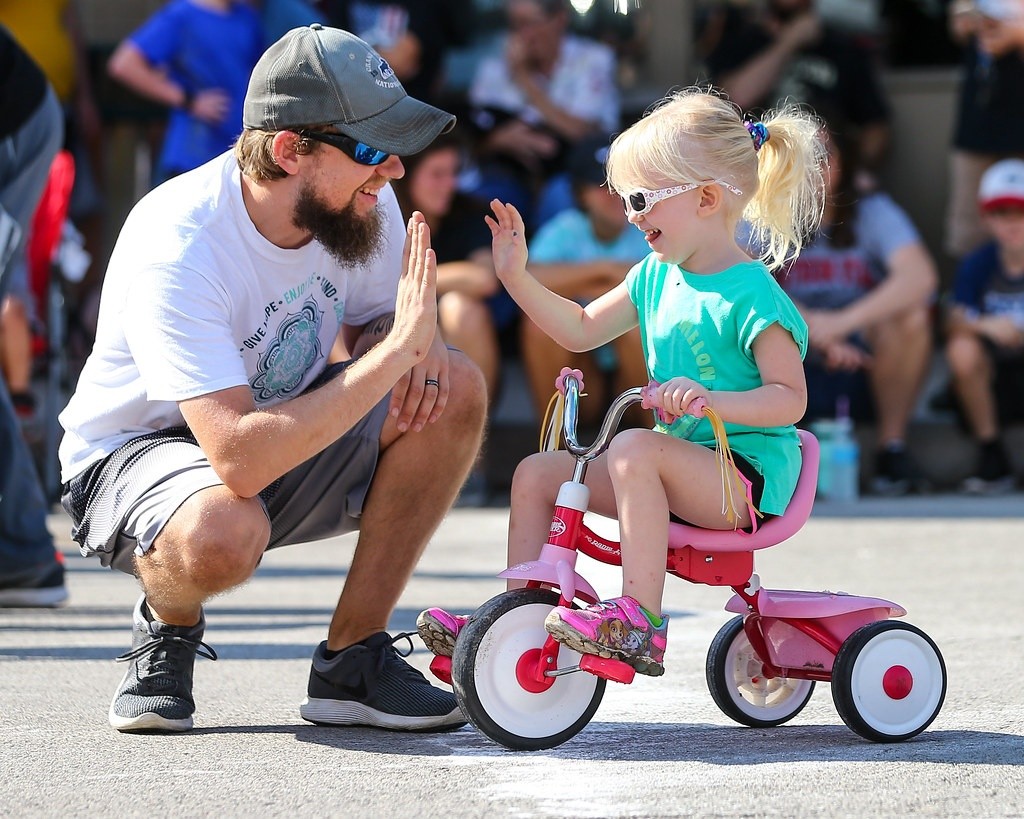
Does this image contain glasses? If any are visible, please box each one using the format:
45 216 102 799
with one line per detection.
294 127 389 166
621 179 743 217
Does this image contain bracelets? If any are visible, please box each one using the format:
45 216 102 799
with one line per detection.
181 88 195 111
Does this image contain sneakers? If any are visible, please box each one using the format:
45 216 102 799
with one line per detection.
417 607 467 657
107 595 206 732
300 631 470 730
543 595 672 677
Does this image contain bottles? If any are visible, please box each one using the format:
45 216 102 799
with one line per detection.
813 421 858 502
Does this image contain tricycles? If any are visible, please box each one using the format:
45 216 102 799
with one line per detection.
429 366 949 751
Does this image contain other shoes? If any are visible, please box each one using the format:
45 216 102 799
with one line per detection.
871 441 918 495
964 440 1016 495
0 565 69 608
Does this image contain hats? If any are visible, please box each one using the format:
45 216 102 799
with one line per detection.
243 24 457 154
976 160 1024 211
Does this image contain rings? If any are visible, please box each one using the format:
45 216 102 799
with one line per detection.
426 380 439 387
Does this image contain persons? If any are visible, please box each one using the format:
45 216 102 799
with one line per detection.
60 23 487 735
0 23 66 606
107 0 1024 497
416 92 826 677
